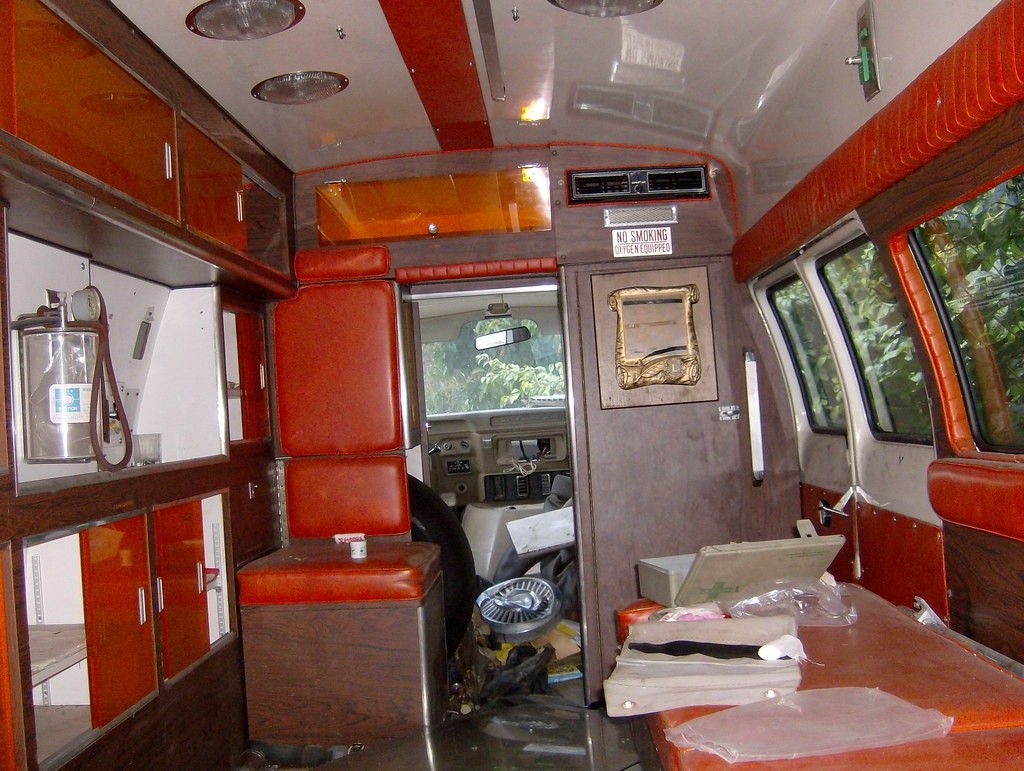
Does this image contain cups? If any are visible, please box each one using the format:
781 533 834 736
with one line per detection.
132 433 162 468
350 540 367 559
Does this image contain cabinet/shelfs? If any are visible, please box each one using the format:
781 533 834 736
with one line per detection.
0 1 297 771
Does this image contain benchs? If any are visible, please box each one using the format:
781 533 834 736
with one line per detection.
616 582 1024 771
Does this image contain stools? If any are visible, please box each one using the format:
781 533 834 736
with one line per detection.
236 542 448 749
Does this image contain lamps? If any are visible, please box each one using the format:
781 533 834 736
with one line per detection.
251 71 349 105
547 0 663 19
185 0 306 40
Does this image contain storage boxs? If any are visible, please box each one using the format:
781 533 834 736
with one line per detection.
637 519 846 607
